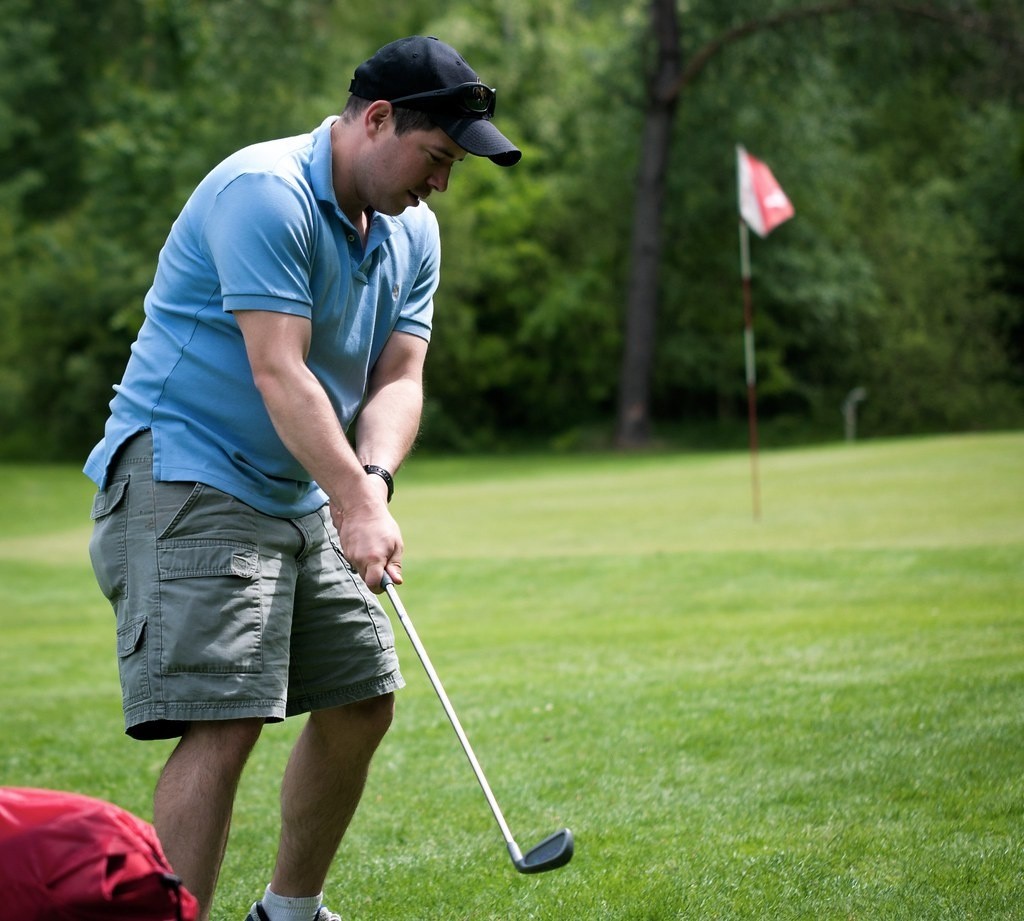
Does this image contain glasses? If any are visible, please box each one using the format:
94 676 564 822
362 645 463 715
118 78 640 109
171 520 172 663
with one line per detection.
388 81 497 119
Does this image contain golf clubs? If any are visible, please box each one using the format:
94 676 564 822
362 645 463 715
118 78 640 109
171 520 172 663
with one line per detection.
381 569 574 873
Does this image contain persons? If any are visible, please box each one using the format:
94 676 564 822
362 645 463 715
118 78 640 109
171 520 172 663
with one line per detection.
82 36 523 921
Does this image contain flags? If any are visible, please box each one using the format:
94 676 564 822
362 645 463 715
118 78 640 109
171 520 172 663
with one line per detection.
740 152 795 238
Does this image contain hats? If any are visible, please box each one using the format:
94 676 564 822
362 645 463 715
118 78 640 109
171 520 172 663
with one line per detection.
348 36 522 167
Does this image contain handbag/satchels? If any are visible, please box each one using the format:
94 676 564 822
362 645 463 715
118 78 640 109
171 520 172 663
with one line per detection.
0 786 200 921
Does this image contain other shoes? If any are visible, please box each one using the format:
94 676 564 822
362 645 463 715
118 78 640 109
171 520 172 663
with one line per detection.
243 902 342 921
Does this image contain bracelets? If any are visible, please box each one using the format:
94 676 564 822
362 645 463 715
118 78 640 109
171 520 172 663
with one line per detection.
363 465 394 503
